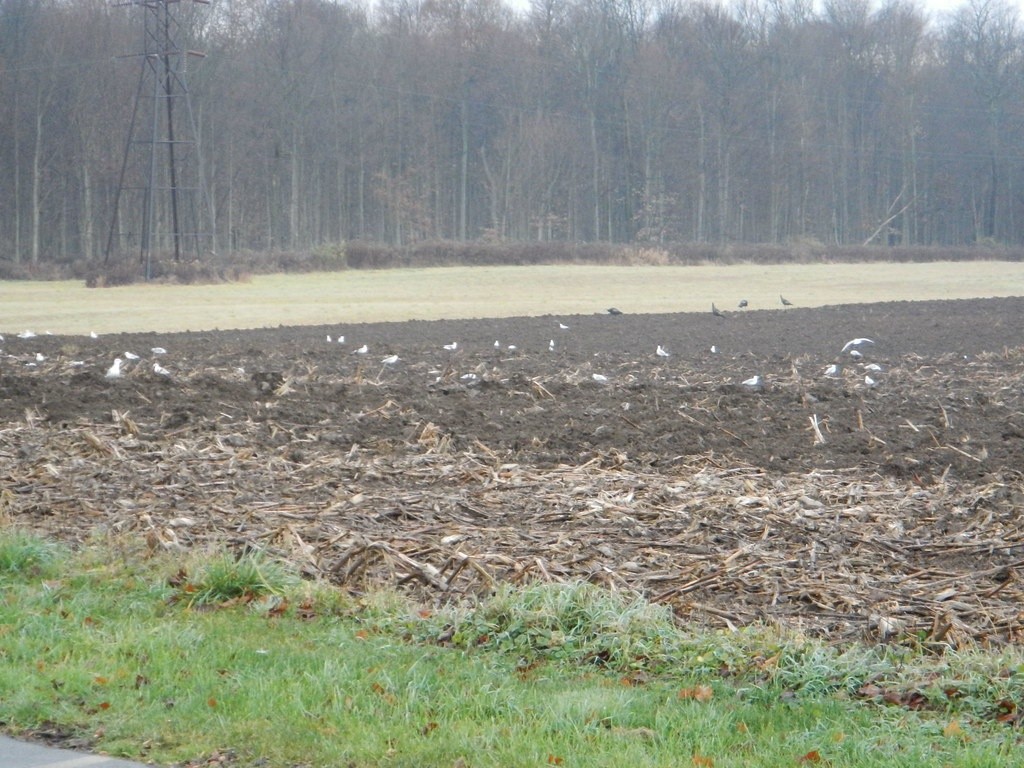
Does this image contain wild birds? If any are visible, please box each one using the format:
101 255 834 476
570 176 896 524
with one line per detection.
151 347 166 354
710 345 717 353
90 329 97 339
592 373 607 381
18 329 35 340
152 363 170 375
35 351 44 362
824 364 836 375
494 339 499 347
839 337 875 359
742 375 760 386
711 302 727 320
327 336 332 342
607 307 623 314
124 351 139 360
105 358 123 377
338 335 345 343
380 355 398 364
655 346 670 357
443 341 458 350
863 364 882 372
354 344 367 353
737 300 749 311
558 323 568 329
778 293 793 309
865 375 874 386
548 338 555 352
